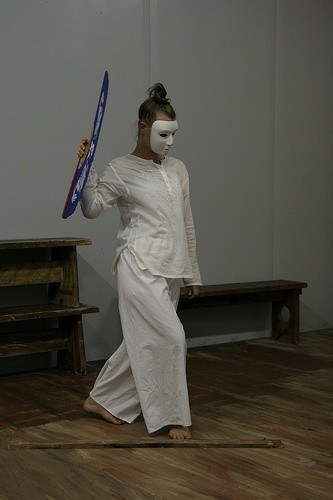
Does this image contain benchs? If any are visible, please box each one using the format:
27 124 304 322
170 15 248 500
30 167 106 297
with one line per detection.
177 280 308 345
0 237 100 376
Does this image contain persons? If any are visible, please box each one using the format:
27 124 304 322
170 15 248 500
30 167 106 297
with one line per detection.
77 83 202 440
